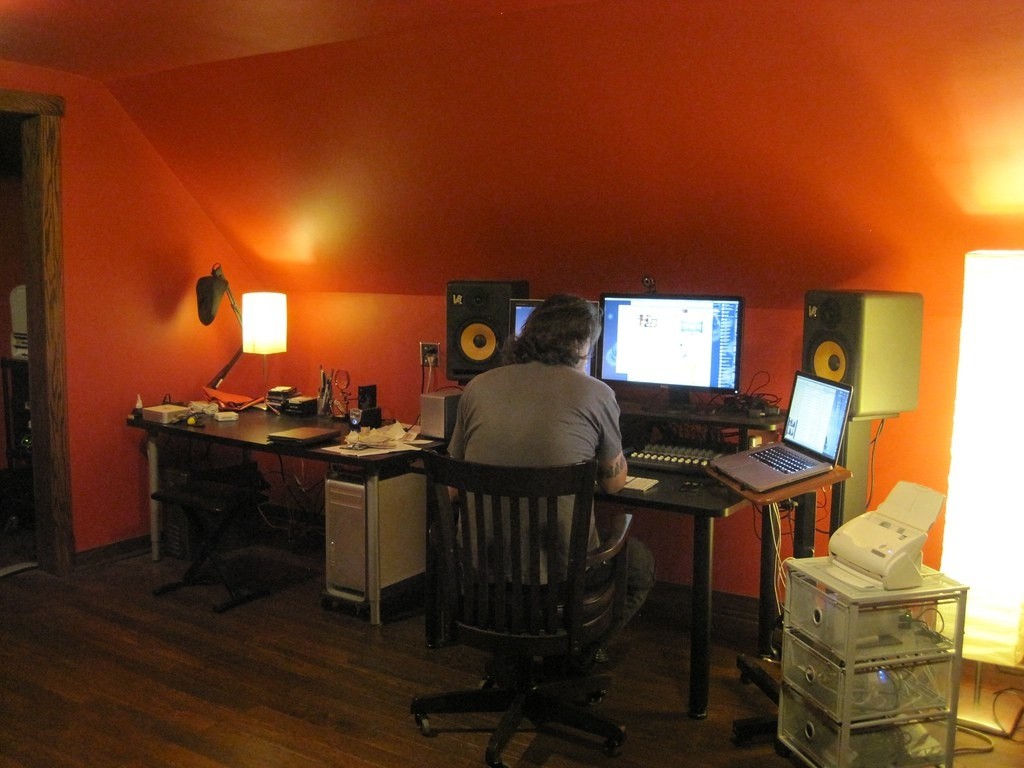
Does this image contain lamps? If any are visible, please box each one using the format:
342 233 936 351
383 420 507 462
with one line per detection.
195 263 245 411
241 291 287 415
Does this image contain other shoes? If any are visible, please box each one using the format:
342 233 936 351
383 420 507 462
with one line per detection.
581 642 610 664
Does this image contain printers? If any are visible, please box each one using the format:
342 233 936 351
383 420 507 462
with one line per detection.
826 481 945 591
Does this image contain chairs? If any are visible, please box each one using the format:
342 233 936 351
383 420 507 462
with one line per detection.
410 443 634 768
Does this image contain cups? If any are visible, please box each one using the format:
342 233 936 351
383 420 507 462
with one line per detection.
316 395 347 420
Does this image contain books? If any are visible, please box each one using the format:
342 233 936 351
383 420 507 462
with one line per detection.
624 475 658 491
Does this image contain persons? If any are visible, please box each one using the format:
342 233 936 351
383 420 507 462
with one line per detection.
448 295 655 702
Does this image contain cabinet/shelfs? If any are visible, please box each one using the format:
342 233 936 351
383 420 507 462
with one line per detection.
777 555 969 768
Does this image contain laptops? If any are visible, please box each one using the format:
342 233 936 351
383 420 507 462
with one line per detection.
711 370 854 493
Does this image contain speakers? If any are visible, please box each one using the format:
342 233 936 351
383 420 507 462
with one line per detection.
803 288 923 421
446 281 528 382
348 384 381 430
419 386 467 441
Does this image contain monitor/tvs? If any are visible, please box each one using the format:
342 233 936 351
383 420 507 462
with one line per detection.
596 293 744 415
507 298 599 380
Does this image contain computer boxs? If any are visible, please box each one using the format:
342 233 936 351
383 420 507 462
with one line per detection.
324 466 428 602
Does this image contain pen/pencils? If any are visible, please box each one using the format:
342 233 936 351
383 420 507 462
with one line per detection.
318 365 334 416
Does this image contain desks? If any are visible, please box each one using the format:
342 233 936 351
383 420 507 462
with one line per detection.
414 449 816 719
122 405 429 624
